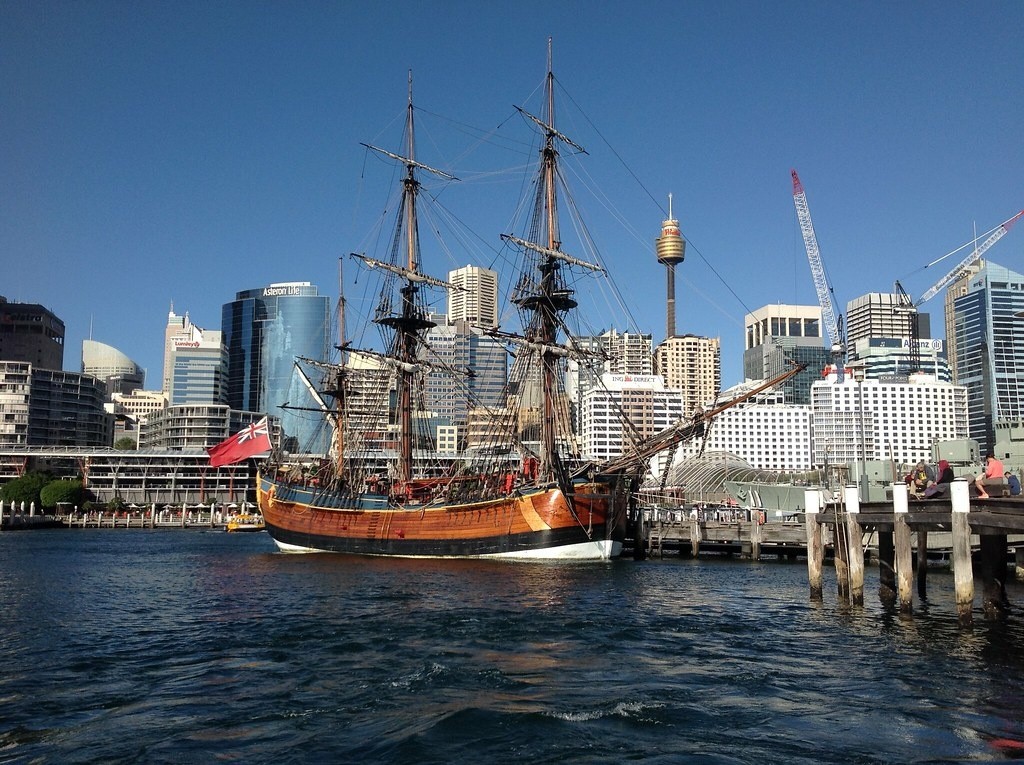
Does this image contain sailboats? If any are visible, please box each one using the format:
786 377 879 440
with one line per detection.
252 35 811 562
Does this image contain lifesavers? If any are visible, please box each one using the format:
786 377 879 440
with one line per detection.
753 512 764 525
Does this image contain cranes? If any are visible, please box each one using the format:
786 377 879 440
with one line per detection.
787 166 847 384
905 210 1024 311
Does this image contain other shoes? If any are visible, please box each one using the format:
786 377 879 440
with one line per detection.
914 495 921 500
978 494 989 498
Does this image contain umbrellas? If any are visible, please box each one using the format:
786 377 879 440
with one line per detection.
228 503 241 513
161 503 194 515
129 503 151 510
241 502 257 513
213 503 223 511
193 502 210 512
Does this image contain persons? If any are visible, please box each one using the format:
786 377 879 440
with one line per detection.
974 451 1004 498
40 508 46 521
717 498 732 523
905 461 935 493
159 507 244 523
1004 472 1021 495
78 509 150 522
914 460 955 501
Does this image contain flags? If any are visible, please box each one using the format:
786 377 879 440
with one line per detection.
206 417 272 468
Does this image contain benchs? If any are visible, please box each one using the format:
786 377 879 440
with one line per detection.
886 484 1011 500
